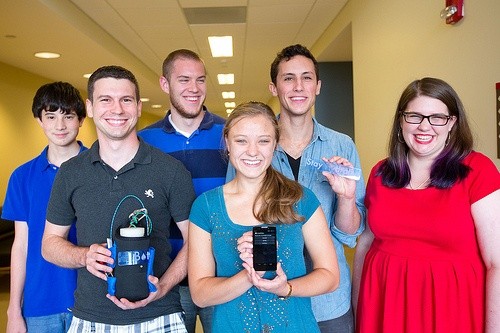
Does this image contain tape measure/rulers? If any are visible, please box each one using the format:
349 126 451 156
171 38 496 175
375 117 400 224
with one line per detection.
303 158 361 180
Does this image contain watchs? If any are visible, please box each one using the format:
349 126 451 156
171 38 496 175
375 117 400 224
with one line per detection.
277 281 292 301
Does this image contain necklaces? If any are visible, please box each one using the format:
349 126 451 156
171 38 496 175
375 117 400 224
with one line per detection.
409 176 430 190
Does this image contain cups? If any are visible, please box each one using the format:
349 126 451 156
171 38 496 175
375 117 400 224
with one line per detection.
116 228 149 301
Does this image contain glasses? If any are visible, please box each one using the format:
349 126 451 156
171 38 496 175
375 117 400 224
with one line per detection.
398 110 451 126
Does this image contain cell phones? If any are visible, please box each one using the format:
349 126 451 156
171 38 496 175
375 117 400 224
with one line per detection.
253 226 277 271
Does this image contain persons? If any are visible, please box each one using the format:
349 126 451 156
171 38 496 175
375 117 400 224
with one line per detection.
351 77 500 333
137 49 230 333
188 101 339 333
0 81 89 333
41 66 197 333
225 43 366 333
